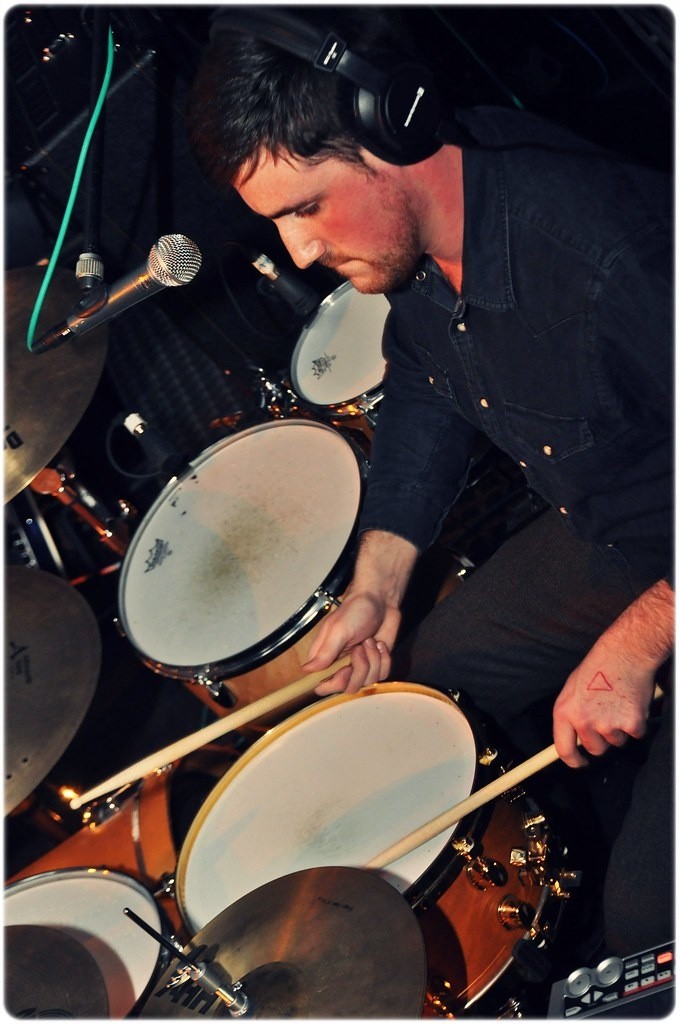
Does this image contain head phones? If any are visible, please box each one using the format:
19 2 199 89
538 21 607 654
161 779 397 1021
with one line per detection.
209 5 453 167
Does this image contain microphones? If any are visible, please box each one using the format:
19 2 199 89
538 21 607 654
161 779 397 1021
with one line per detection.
246 248 319 316
121 411 188 478
32 234 202 356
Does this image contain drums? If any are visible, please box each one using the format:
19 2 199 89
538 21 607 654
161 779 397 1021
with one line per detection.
174 679 550 1011
6 869 190 1022
112 416 414 734
291 275 431 451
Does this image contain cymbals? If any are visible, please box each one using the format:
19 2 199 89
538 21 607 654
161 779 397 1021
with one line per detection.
6 924 114 1021
127 865 431 1019
6 261 109 515
7 555 102 816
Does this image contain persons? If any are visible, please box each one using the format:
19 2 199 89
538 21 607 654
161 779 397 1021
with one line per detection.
198 4 675 958
